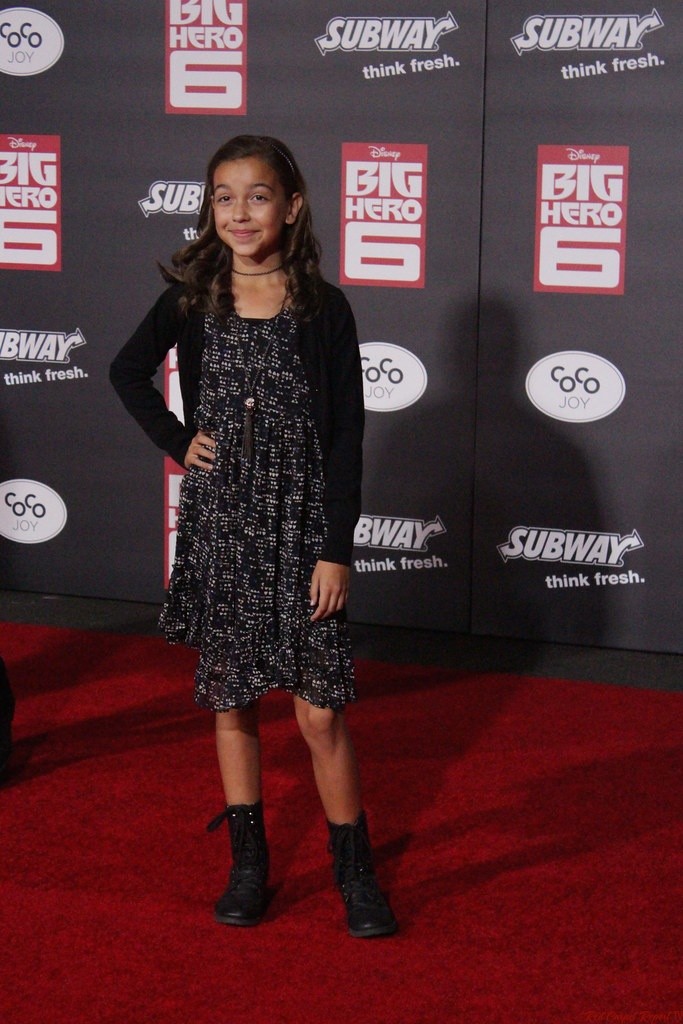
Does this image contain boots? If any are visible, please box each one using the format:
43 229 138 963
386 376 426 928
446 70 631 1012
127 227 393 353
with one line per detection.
326 811 397 936
206 800 270 926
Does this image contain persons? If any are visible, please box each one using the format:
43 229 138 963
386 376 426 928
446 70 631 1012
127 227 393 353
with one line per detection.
110 135 399 937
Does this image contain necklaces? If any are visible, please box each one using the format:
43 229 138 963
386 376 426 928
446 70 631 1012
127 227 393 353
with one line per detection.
231 263 283 276
